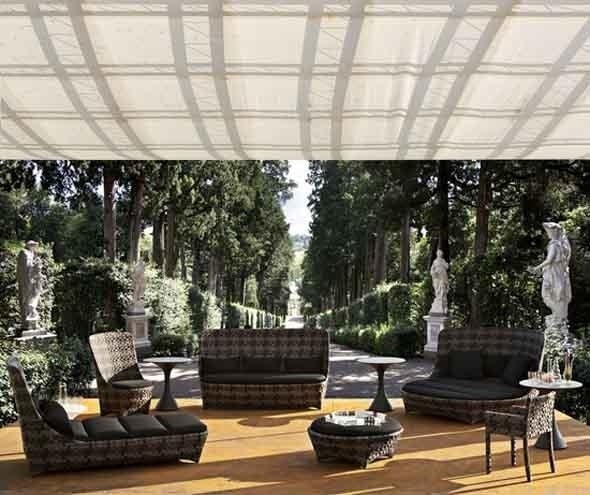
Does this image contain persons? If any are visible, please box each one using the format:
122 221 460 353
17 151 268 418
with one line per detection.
530 221 572 317
133 258 146 301
430 249 449 298
32 262 45 294
18 240 46 316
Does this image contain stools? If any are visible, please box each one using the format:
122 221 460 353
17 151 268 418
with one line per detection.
307 410 404 469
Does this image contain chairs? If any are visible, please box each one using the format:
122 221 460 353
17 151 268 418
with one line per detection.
484 391 557 480
6 355 208 473
89 331 154 416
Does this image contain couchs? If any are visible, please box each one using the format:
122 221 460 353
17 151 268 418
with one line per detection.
198 326 330 410
400 328 545 425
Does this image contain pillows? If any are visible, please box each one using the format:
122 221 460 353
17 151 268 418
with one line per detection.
489 356 525 386
449 350 485 379
40 398 74 434
251 356 283 373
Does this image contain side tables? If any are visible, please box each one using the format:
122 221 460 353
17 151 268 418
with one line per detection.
519 377 583 450
356 356 407 413
142 357 192 410
